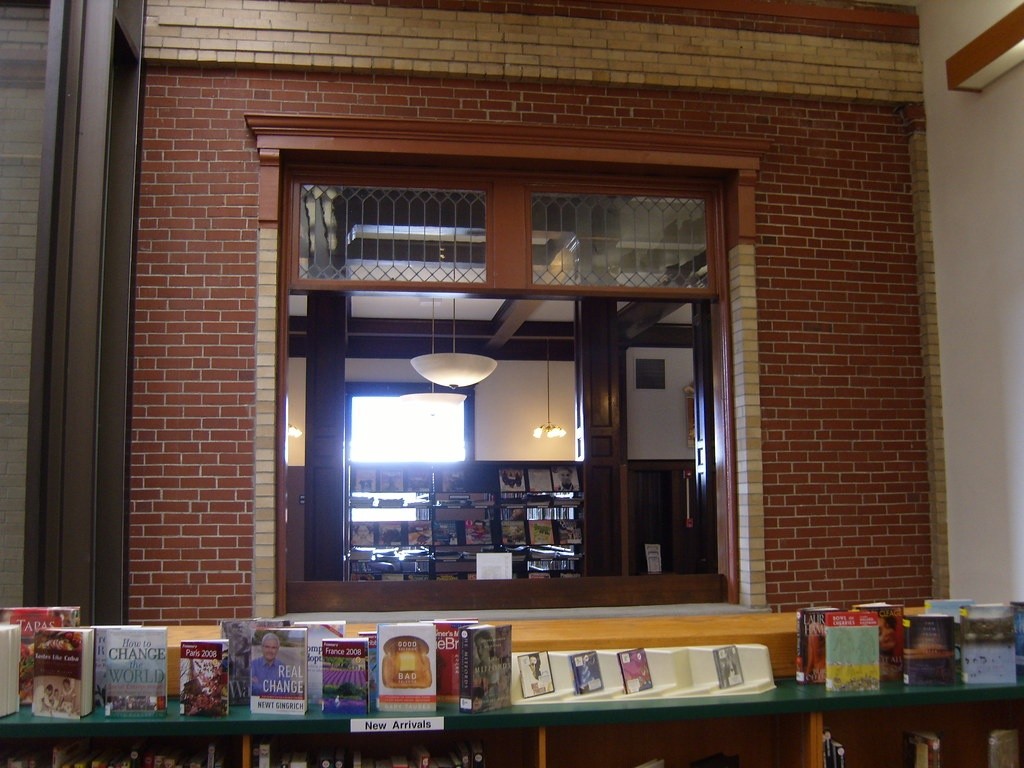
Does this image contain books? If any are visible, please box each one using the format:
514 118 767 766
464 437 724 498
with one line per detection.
105 625 168 719
179 618 513 716
517 650 555 699
616 647 654 695
32 627 97 719
90 624 145 709
569 651 605 696
0 607 80 706
822 727 846 768
713 645 744 690
350 464 583 583
904 730 941 768
796 599 1024 692
0 625 22 718
6 743 486 768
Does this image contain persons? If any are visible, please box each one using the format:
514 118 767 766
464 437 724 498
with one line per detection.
252 633 291 695
555 468 574 491
529 653 546 694
352 523 372 545
44 679 75 713
878 615 901 680
474 630 502 696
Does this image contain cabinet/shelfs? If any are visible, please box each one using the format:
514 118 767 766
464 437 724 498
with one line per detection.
0 677 1024 768
344 460 584 581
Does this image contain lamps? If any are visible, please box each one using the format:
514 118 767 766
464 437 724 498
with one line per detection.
533 339 566 438
397 299 466 418
410 296 498 389
